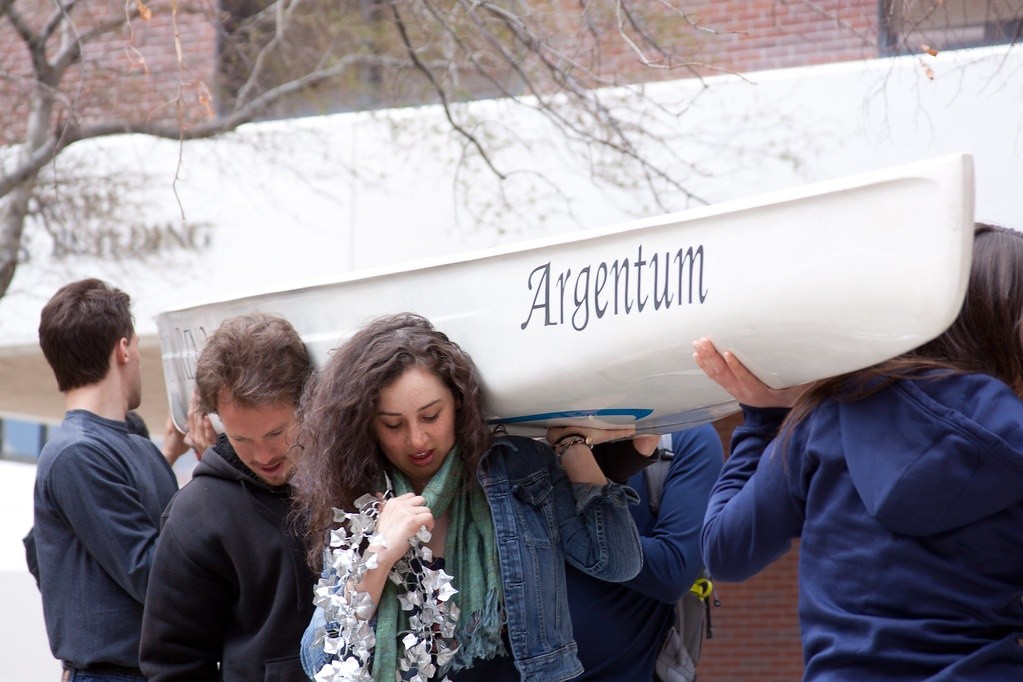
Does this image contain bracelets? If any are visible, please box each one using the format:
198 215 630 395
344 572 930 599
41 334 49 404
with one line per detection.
553 432 593 470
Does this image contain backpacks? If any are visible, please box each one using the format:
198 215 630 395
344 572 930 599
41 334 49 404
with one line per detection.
642 434 720 682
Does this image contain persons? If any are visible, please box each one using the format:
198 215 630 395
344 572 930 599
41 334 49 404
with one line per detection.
566 423 724 682
693 222 1023 682
291 312 644 682
137 316 322 682
23 277 217 682
